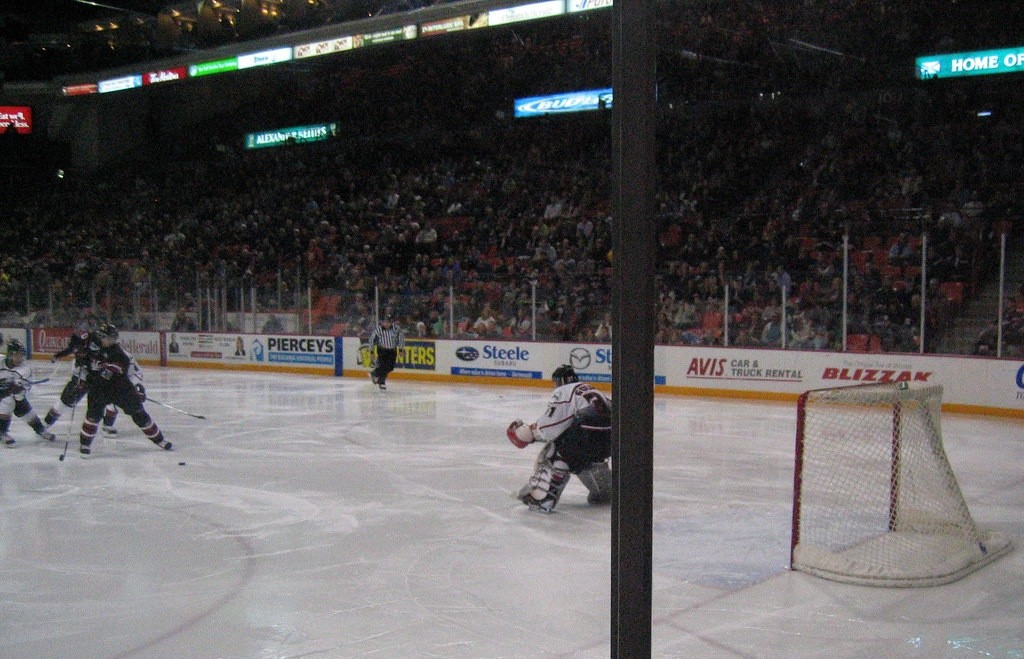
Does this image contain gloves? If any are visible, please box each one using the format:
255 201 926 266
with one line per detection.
134 382 146 401
97 369 113 388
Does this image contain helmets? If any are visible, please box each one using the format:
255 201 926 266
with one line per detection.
7 340 25 353
552 365 579 387
96 324 119 339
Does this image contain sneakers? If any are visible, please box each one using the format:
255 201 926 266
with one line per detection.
0 431 16 448
38 429 58 442
80 442 91 459
156 440 174 451
102 424 118 438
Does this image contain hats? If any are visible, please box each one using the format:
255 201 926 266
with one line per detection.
384 314 393 320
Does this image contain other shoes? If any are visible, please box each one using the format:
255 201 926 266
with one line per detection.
369 373 377 385
379 383 386 390
523 495 553 512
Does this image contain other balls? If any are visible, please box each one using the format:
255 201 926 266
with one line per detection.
179 462 185 465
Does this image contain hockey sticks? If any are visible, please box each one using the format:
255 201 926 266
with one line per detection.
18 356 64 386
136 395 208 419
58 357 85 461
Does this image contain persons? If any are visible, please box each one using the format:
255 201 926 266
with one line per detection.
0 0 1024 360
235 336 245 356
506 365 612 514
369 316 405 391
169 334 179 353
43 314 176 458
0 340 56 447
